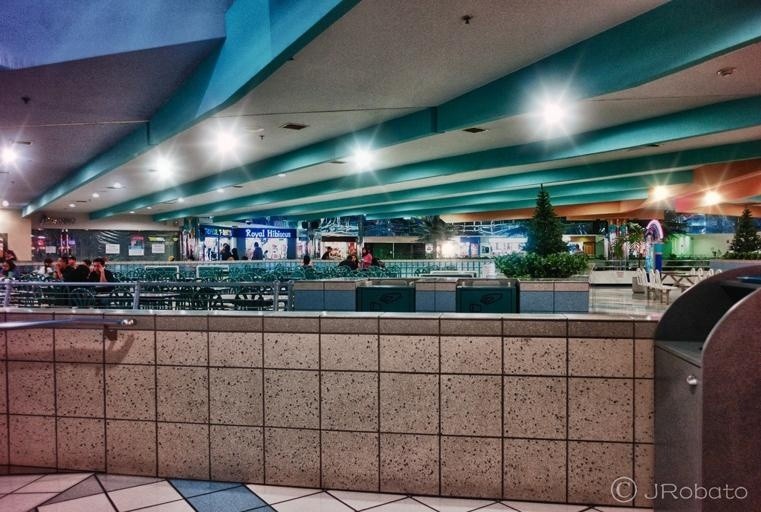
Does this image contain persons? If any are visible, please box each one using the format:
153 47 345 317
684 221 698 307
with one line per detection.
1 261 20 279
323 247 333 260
3 249 17 260
301 255 312 269
574 244 582 252
205 242 250 260
251 242 263 261
332 244 386 271
39 259 53 273
263 244 288 260
53 254 115 308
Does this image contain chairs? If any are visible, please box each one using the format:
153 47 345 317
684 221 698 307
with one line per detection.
2 262 457 310
635 266 722 305
